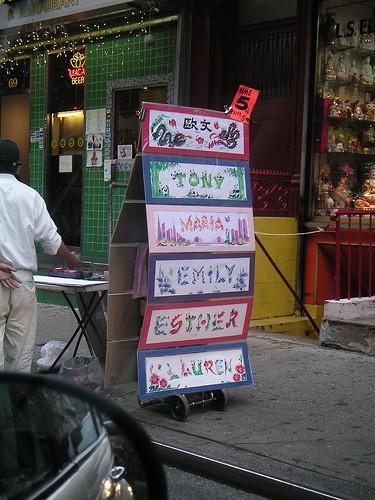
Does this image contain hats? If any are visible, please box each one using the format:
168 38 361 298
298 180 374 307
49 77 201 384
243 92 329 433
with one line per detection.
0 140 20 165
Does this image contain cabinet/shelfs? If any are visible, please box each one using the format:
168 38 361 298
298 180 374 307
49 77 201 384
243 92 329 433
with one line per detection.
301 3 374 317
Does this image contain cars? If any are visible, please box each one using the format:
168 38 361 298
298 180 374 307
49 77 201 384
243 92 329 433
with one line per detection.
0 371 167 500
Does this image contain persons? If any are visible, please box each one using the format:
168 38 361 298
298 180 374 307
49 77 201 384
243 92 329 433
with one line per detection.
315 28 375 216
0 139 83 376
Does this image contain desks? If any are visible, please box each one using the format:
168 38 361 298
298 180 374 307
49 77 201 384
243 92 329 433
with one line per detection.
31 275 108 385
